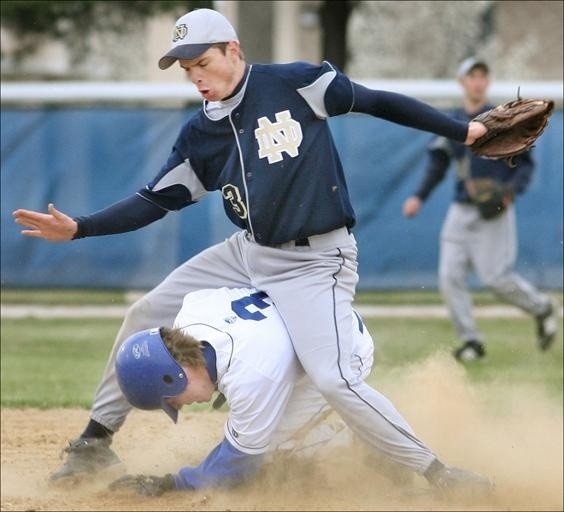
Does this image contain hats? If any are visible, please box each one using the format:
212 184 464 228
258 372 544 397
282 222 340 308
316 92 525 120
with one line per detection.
459 57 487 78
158 8 238 70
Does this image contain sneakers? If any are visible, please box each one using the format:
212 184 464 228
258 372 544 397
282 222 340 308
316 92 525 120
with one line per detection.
430 466 491 506
454 342 484 362
51 437 125 484
536 298 558 348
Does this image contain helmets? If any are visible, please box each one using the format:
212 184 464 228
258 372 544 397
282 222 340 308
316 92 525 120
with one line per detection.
115 327 188 422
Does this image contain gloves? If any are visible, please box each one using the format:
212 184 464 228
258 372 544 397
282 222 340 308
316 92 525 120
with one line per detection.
476 185 513 218
111 475 175 499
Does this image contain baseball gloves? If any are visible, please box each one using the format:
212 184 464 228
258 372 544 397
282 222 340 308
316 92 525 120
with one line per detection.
470 97 554 160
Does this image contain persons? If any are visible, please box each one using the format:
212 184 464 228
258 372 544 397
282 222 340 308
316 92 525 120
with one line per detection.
106 286 416 499
402 55 562 364
12 7 554 499
